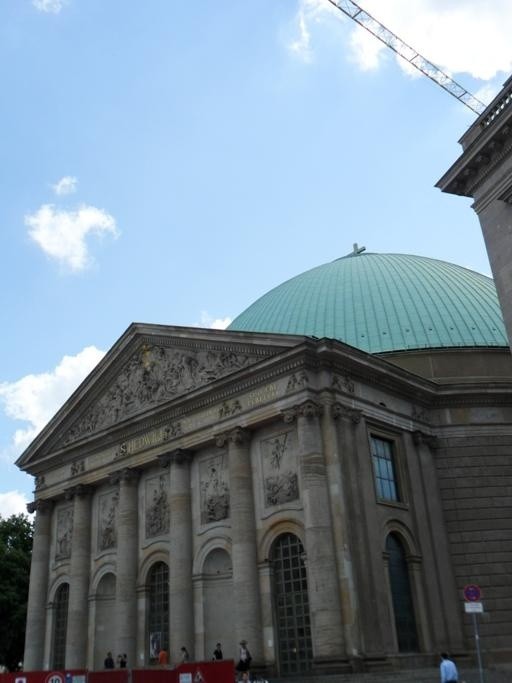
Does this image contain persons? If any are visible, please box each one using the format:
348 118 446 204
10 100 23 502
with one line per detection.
158 648 167 665
439 652 458 683
119 654 127 668
235 639 252 671
178 646 192 663
213 643 223 660
238 672 251 683
104 651 114 668
